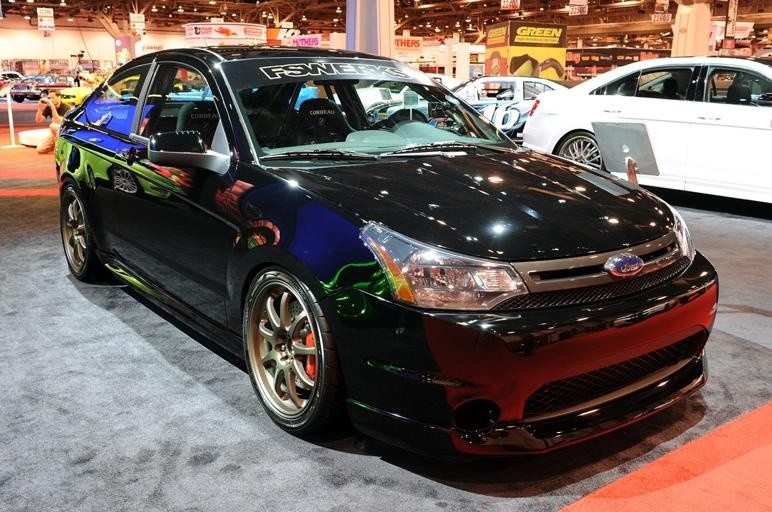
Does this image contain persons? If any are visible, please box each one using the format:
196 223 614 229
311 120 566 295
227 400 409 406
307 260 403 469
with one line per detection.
34 87 71 154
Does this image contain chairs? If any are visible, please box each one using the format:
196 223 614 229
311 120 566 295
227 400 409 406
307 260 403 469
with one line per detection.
176 98 356 148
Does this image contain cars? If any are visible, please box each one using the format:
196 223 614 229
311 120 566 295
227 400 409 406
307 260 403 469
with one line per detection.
52 48 720 463
0 68 197 124
522 55 772 210
446 75 570 144
